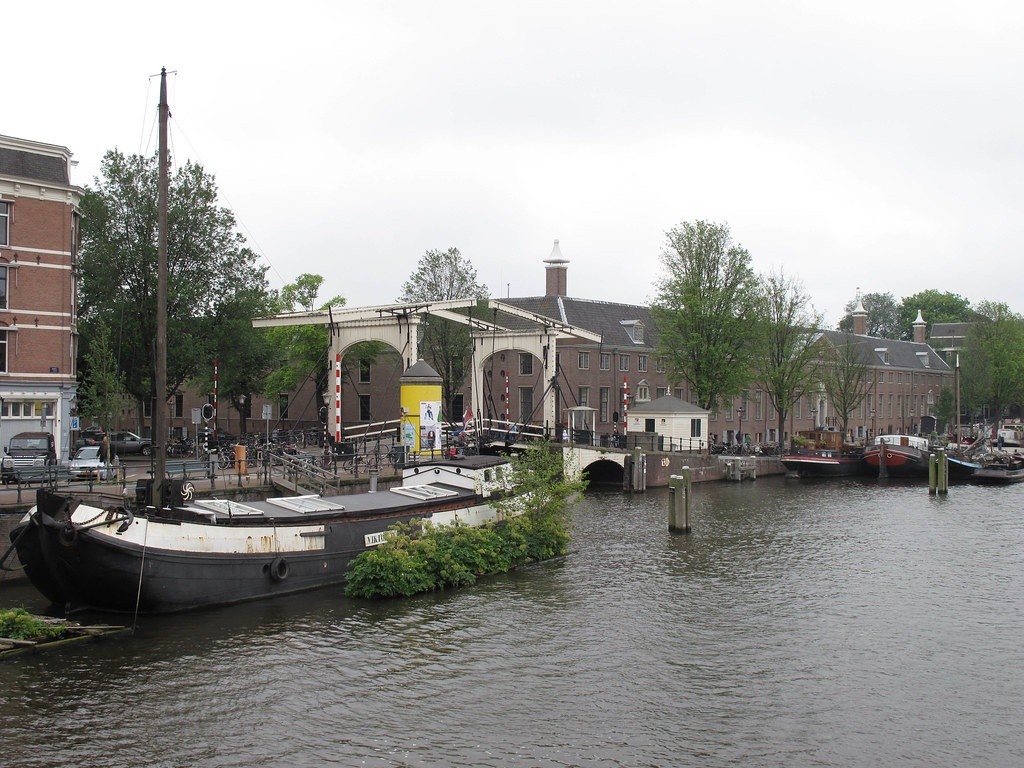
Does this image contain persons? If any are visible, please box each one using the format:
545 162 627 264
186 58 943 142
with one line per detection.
1013 449 1022 455
97 437 114 480
709 433 715 448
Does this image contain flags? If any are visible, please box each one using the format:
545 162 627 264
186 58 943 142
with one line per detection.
463 402 473 423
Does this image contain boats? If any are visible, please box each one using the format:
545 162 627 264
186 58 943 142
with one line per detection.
780 436 867 480
0 60 555 619
863 351 1024 485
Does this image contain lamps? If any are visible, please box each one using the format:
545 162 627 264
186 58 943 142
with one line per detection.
50 367 59 373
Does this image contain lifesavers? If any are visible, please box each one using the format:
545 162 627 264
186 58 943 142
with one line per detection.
55 521 77 548
270 556 290 582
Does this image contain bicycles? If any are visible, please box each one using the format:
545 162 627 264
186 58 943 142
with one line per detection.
706 436 785 457
165 428 403 481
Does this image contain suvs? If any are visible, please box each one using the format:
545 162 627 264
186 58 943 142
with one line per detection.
73 427 153 456
0 432 57 484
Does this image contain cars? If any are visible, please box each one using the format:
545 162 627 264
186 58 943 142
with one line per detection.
68 445 121 482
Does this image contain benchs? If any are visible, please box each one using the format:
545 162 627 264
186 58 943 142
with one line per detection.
13 465 75 490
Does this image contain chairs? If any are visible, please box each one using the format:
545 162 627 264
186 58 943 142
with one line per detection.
15 440 28 448
38 440 48 448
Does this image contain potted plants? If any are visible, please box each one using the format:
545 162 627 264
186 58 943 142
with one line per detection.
792 434 816 450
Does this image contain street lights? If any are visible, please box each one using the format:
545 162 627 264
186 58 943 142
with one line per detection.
910 409 916 435
870 408 877 439
236 394 249 445
736 406 744 455
810 407 818 442
